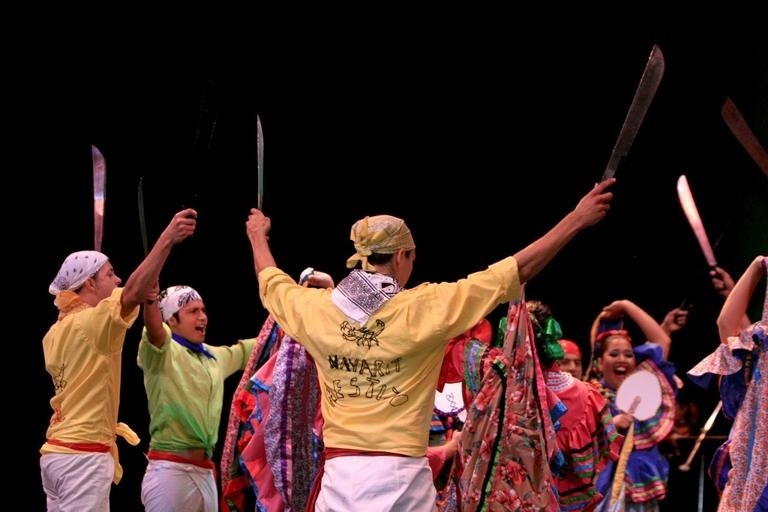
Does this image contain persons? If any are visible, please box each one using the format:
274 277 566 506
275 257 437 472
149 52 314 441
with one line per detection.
135 275 257 511
302 254 768 512
245 177 619 511
41 208 199 512
